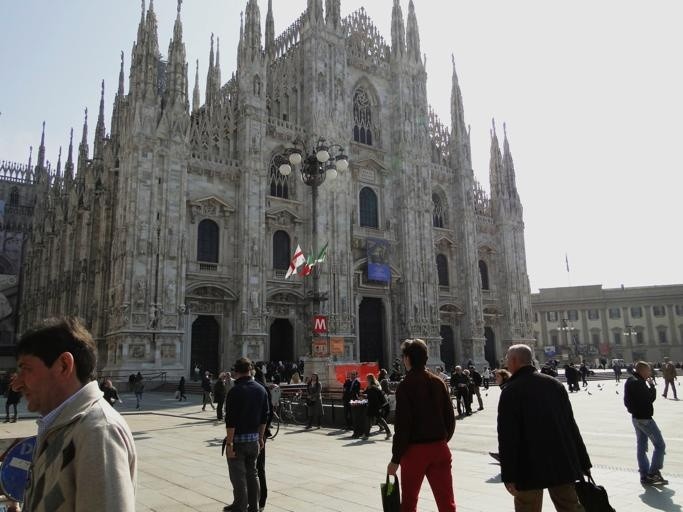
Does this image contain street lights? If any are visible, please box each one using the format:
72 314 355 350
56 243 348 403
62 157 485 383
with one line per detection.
621 324 638 362
557 319 575 348
278 136 352 338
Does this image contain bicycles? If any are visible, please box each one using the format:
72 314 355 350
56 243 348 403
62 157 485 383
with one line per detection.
446 394 464 420
271 389 313 426
263 408 281 440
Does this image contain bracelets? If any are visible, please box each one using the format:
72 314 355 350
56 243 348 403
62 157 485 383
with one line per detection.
224 442 233 446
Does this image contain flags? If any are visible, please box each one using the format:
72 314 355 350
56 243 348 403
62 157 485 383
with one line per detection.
284 244 305 279
301 245 316 276
314 242 329 265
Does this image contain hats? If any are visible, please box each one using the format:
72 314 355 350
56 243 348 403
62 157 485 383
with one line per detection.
234 357 251 373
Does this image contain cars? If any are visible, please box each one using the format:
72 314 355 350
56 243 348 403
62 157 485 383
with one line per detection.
571 357 629 369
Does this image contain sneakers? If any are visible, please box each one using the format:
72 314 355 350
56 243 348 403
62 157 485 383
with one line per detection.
575 471 616 512
640 471 668 483
224 504 245 511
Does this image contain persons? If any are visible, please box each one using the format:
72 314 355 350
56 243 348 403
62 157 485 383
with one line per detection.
12 314 139 511
496 343 593 512
623 360 668 485
133 379 144 409
541 355 590 393
176 376 186 401
612 362 621 382
128 373 135 390
3 373 21 424
100 379 122 406
384 336 457 511
660 356 678 399
221 357 270 511
196 356 490 441
245 364 274 512
486 369 512 461
133 371 142 382
